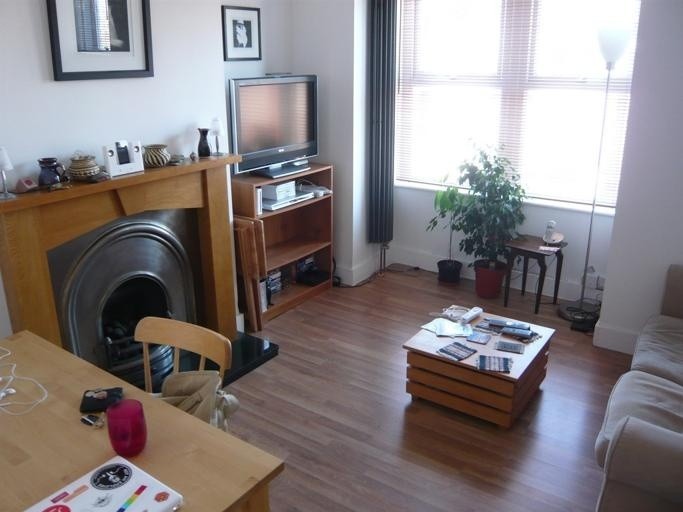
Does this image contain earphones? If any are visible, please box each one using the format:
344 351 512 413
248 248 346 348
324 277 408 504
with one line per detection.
0 391 6 401
6 388 16 394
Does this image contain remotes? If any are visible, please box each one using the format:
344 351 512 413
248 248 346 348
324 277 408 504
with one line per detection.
488 320 529 330
499 327 533 339
291 159 307 166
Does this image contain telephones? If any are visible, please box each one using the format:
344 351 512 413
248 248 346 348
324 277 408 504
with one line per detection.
543 220 564 244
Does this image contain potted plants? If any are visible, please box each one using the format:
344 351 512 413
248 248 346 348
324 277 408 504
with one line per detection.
425 150 526 299
434 186 466 282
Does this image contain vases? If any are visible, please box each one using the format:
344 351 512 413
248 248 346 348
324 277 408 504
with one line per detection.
197 127 211 157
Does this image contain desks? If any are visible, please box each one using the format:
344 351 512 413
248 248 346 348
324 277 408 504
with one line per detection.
0 330 285 511
504 234 568 315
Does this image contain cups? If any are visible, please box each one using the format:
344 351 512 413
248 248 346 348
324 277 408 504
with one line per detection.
106 399 147 458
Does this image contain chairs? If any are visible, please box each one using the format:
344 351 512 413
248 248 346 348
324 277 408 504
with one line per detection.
134 317 233 394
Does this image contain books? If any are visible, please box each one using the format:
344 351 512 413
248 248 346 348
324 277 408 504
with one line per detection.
268 270 283 294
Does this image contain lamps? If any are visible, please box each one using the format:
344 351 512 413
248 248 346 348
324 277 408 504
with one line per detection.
208 119 227 156
0 146 15 199
558 29 626 321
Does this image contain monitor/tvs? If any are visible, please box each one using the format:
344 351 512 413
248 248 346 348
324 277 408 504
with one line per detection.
227 74 319 179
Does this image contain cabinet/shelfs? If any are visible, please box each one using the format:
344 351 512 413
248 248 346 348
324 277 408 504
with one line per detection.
231 162 334 324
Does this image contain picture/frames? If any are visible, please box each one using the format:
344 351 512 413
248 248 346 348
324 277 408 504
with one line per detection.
221 6 263 61
46 0 154 81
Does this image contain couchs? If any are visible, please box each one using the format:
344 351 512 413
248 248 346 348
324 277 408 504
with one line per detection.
595 264 682 512
